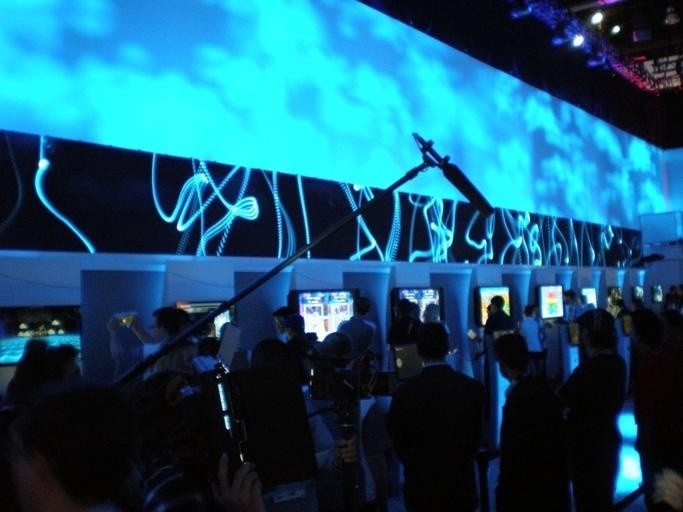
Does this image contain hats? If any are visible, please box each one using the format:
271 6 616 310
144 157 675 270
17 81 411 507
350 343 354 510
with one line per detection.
314 332 354 362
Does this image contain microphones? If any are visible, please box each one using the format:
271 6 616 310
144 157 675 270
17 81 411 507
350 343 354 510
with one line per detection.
413 130 495 217
334 373 361 484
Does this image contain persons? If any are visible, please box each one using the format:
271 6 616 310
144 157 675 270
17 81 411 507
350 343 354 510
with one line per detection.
1 284 683 512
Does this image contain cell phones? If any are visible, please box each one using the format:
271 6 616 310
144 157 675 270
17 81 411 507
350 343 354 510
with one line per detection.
115 311 138 329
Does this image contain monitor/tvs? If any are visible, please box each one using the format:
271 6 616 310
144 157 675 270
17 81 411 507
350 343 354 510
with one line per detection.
535 282 568 322
580 286 597 310
287 287 360 346
632 285 645 304
472 284 514 328
390 284 447 333
653 285 663 303
